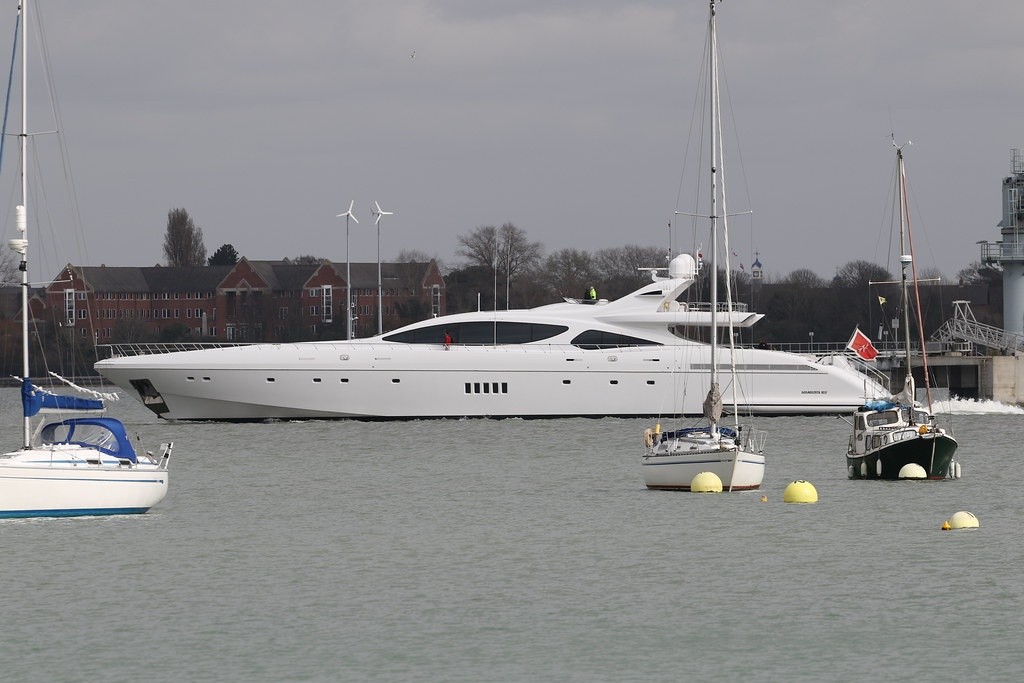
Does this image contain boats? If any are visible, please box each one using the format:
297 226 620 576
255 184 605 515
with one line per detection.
92 246 892 425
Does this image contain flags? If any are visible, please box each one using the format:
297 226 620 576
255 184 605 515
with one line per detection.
847 328 878 361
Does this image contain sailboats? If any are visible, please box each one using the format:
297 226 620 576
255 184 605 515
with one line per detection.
645 0 771 493
844 131 960 481
0 4 174 520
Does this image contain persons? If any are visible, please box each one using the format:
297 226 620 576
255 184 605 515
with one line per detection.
959 278 963 288
443 331 451 351
590 287 597 300
758 339 769 349
